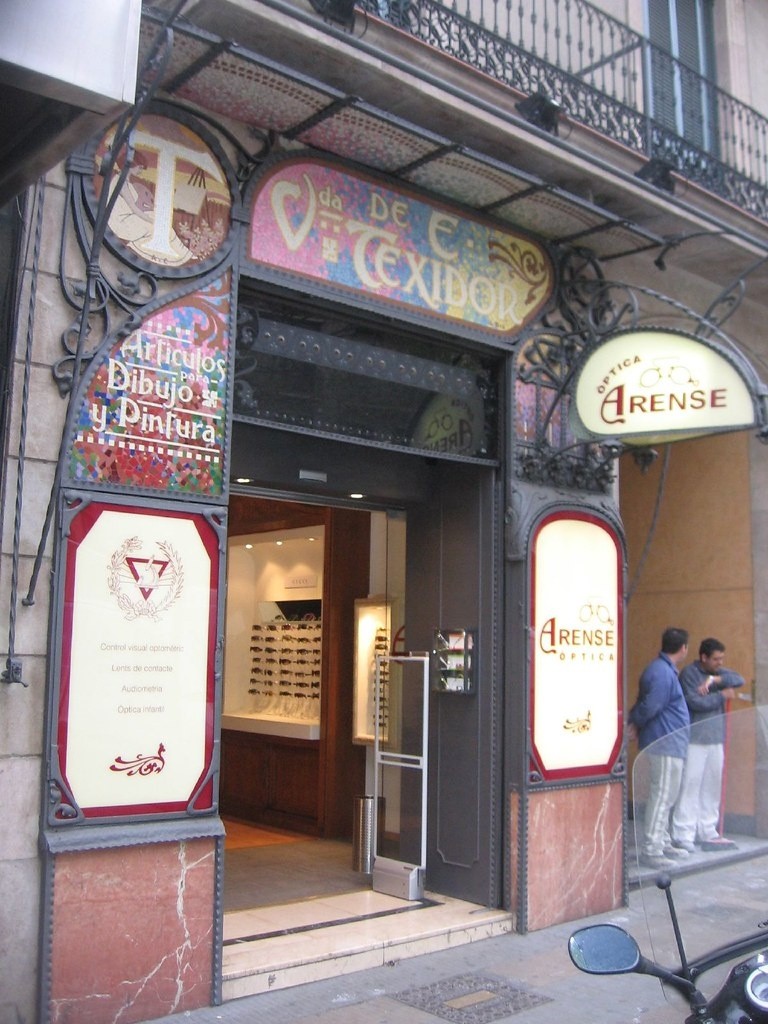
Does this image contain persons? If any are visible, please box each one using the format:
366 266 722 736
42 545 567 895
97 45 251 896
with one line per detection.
627 628 689 871
671 638 745 853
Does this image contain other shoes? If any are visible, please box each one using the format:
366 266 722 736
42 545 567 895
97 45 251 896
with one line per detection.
698 835 734 847
640 852 678 871
673 838 696 853
663 845 688 860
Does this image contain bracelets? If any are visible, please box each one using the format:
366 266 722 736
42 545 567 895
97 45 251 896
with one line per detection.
709 675 715 685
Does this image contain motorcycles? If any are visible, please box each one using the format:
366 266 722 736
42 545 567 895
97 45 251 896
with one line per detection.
566 705 768 1024
